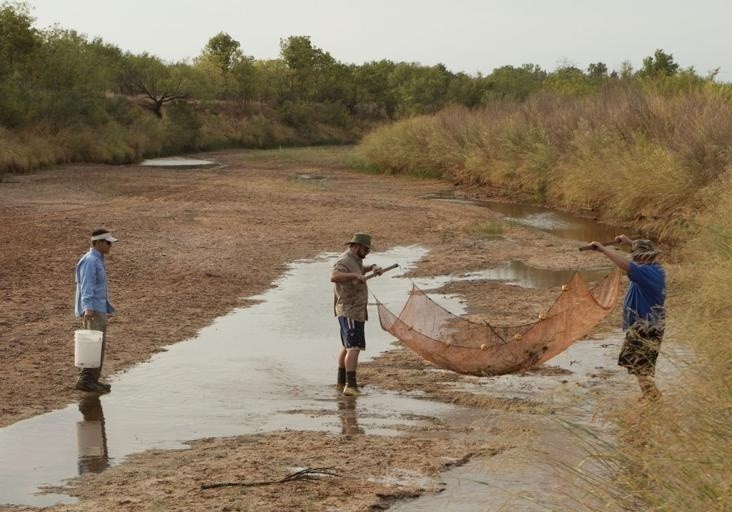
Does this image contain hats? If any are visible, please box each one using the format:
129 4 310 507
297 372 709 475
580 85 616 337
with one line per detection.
628 238 661 259
91 232 118 244
345 232 378 253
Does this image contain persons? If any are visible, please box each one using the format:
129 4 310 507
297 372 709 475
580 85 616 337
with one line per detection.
331 232 382 395
75 229 119 392
588 234 665 402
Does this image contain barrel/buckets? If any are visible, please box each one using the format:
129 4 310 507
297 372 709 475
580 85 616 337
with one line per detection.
72 312 104 371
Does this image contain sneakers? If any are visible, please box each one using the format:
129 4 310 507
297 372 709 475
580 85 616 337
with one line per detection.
335 382 368 396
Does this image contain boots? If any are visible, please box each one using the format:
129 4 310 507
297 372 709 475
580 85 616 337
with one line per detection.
77 368 112 393
